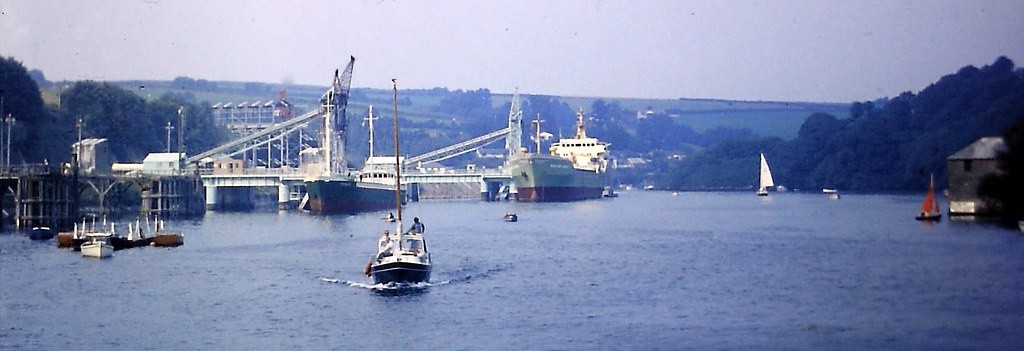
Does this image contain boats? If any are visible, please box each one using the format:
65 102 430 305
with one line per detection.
30 225 55 240
505 214 516 222
507 104 610 203
80 239 114 259
58 216 154 250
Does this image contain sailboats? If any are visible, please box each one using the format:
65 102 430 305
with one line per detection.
363 75 437 287
914 170 942 221
755 152 774 197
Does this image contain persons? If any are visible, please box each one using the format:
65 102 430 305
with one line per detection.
382 230 394 254
403 217 425 251
44 157 50 174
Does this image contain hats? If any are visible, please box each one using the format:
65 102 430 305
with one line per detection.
384 230 389 234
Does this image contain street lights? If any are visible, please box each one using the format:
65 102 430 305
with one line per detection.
164 121 175 154
5 113 16 168
75 118 87 169
